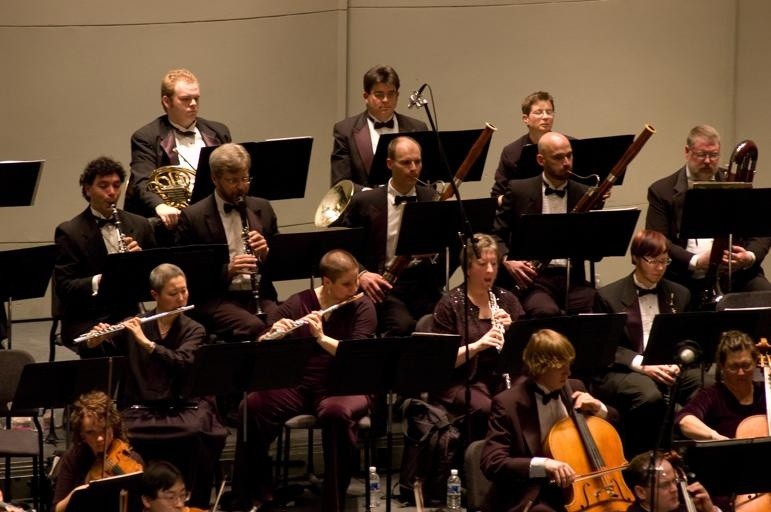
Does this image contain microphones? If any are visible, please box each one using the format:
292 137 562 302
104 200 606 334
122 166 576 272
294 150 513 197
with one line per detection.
407 81 428 109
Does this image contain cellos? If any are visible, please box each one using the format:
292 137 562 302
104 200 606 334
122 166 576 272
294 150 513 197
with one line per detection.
542 372 638 512
731 340 770 512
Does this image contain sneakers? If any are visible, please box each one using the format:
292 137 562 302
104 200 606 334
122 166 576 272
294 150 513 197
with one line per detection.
544 181 568 199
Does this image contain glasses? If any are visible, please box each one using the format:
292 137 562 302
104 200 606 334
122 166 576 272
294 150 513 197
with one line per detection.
646 476 681 491
640 255 674 266
221 174 254 185
530 111 558 118
151 490 193 506
691 148 721 162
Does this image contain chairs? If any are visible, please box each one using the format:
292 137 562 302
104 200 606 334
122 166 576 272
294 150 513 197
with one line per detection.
463 440 490 511
276 413 326 490
0 347 46 511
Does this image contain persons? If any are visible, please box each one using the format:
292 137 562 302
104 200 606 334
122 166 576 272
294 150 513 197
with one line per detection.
124 68 231 229
491 133 604 314
492 91 580 208
49 227 769 512
52 155 159 360
173 142 280 342
343 134 461 337
330 64 437 189
645 125 770 301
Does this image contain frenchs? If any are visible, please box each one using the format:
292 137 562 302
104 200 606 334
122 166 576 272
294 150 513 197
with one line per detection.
147 147 203 213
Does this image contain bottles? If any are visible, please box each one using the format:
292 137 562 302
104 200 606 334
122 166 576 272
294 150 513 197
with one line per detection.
595 272 602 288
446 468 462 509
370 467 382 508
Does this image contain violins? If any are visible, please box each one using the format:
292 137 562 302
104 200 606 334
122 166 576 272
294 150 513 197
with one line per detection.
89 438 142 481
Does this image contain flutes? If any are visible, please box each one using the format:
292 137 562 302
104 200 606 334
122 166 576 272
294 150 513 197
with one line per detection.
258 291 365 340
74 302 194 343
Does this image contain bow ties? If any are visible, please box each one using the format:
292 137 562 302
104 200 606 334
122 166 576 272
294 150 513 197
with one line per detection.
98 216 120 229
175 128 198 138
368 116 396 130
533 383 562 405
635 285 660 297
394 194 419 206
223 200 247 215
